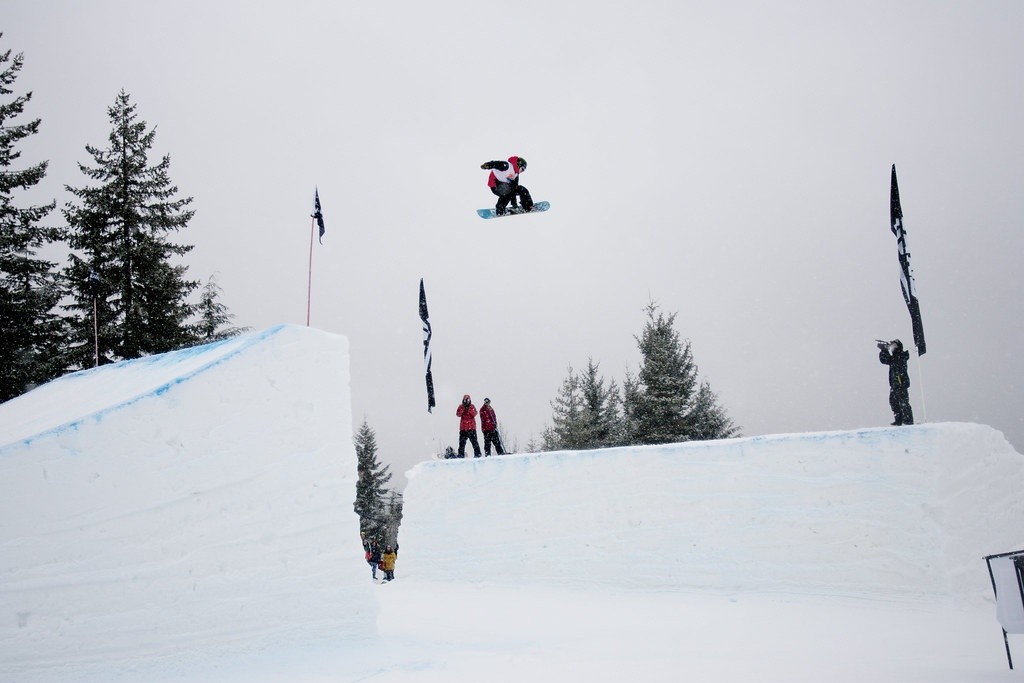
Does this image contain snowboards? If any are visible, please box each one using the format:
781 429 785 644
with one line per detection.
475 200 550 219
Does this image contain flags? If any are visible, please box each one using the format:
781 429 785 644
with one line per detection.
310 186 326 245
984 548 1024 635
890 164 927 357
418 278 437 415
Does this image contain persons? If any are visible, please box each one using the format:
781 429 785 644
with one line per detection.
455 394 482 458
479 397 507 457
364 539 381 579
480 155 535 216
878 338 915 426
382 544 397 581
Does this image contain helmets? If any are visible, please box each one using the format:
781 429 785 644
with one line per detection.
517 157 527 169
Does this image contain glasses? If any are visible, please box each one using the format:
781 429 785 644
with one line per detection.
522 168 524 171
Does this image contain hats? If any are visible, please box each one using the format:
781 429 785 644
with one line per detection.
891 339 903 352
